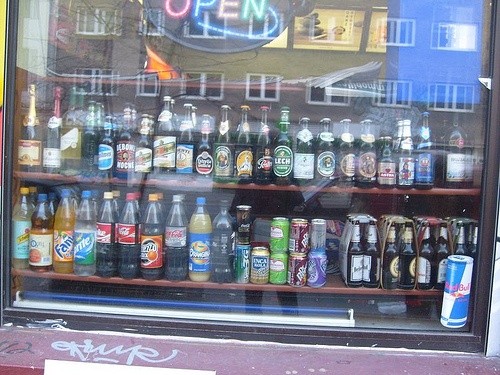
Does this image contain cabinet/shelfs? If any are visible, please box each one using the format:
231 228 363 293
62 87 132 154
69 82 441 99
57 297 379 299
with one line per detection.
7 76 481 322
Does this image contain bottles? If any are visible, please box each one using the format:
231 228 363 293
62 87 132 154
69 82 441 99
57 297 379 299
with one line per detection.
10 184 236 284
16 85 483 190
338 212 480 290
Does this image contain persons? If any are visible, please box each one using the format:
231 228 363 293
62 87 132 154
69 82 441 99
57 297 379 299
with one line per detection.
397 154 473 318
229 126 307 316
